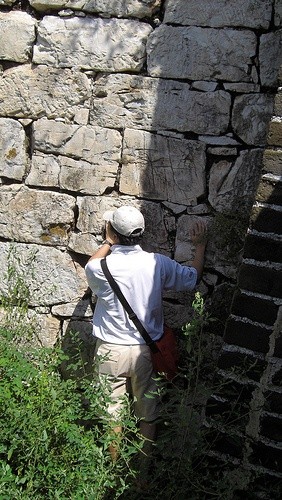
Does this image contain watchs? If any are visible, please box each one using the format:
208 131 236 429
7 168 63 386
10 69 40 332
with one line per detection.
101 240 113 248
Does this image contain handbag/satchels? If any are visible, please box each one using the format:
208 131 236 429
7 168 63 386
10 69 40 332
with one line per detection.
149 324 178 379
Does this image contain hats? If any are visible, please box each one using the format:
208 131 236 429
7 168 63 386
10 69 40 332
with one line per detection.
102 206 145 237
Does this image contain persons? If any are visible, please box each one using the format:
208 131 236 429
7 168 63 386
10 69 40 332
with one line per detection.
84 205 207 490
